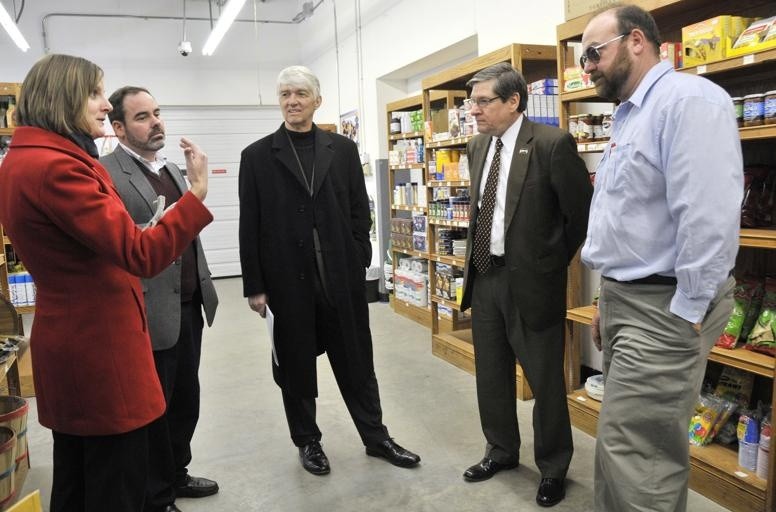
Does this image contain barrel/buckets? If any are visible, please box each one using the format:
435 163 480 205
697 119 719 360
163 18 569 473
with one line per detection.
383 260 394 290
1 396 30 511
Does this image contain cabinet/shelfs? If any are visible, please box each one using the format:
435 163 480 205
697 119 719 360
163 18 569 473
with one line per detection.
419 41 579 401
383 90 467 336
0 238 35 508
553 2 776 512
1 80 42 403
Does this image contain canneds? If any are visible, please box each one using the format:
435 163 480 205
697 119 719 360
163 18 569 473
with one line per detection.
390 118 401 135
568 113 613 143
731 90 776 128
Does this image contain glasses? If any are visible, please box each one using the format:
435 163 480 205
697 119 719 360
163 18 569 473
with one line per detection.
579 30 632 72
466 94 506 108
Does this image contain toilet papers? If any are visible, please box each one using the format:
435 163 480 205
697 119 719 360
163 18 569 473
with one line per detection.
399 257 428 273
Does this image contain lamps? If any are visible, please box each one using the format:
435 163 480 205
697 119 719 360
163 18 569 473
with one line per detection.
0 1 30 56
198 1 245 56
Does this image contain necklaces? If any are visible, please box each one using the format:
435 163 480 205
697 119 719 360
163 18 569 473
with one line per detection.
287 131 315 197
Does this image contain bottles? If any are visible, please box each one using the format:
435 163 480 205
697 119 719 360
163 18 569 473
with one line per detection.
392 181 418 206
388 115 401 132
428 188 469 219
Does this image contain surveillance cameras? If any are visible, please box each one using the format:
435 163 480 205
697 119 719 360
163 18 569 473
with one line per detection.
177 41 192 57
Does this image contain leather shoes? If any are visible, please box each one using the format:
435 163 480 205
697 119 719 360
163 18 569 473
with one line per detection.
463 453 520 483
535 477 567 507
163 503 183 512
177 474 220 499
297 440 333 476
362 436 422 468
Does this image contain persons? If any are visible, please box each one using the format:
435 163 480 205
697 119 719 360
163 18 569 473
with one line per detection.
460 61 594 507
579 3 745 512
98 87 220 511
239 62 420 476
1 55 214 511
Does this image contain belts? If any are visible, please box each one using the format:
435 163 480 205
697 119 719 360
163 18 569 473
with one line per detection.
489 252 505 268
601 272 678 287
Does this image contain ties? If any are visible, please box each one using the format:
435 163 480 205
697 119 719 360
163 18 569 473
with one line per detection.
469 136 505 277
